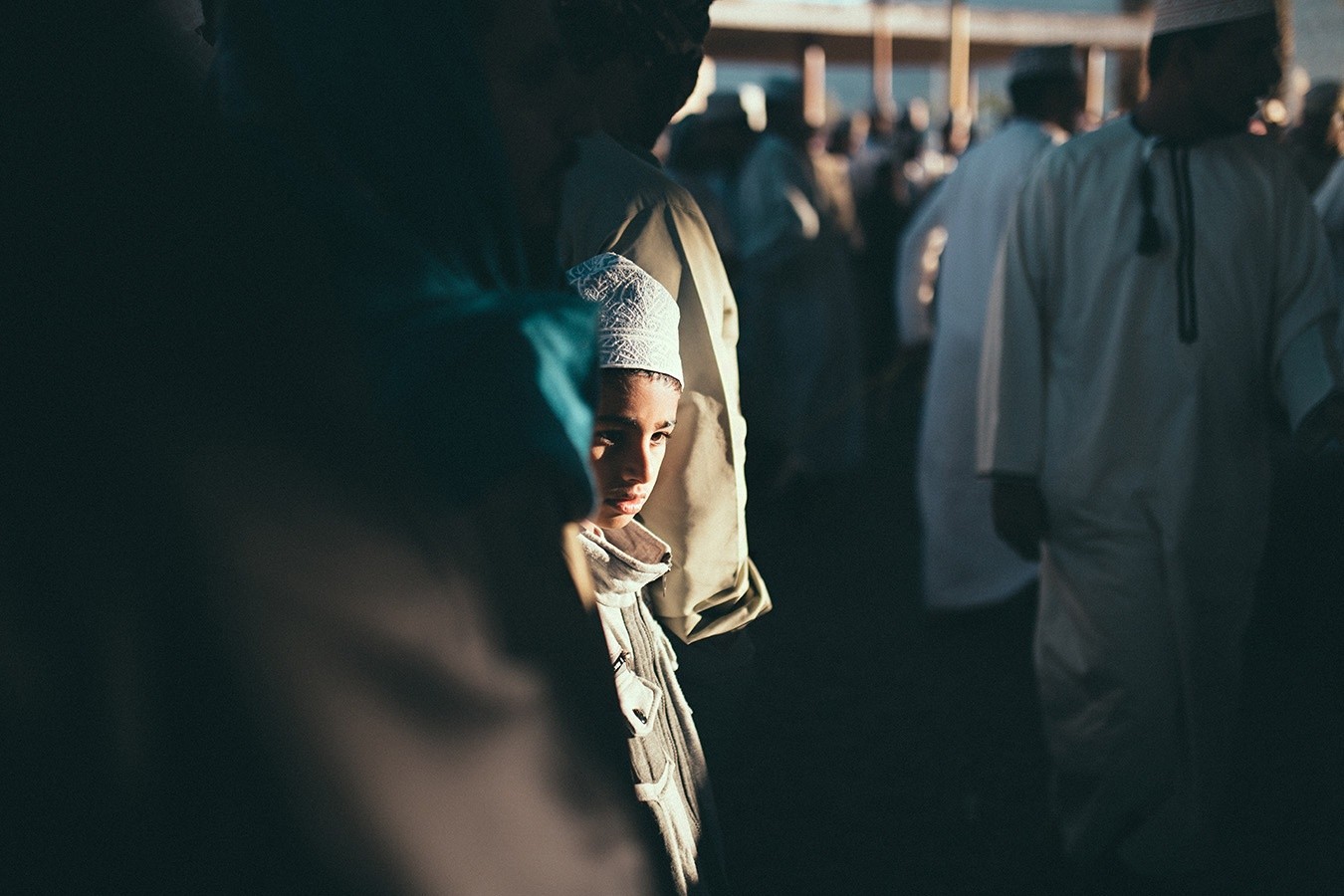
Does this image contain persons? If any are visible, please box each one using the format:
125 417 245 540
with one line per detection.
0 1 1344 896
561 251 712 896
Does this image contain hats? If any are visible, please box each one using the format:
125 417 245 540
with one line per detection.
564 252 684 393
1153 0 1275 37
